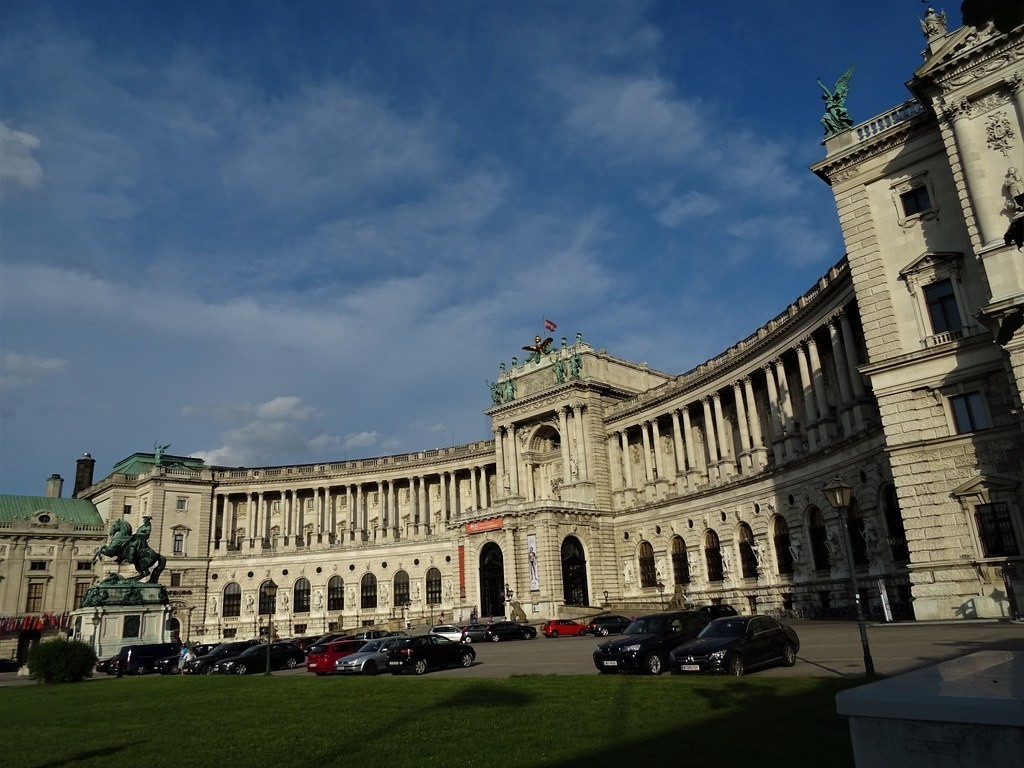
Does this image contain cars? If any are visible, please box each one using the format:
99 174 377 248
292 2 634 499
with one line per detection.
333 634 409 675
182 640 261 674
272 629 408 675
210 641 305 675
588 613 634 637
669 614 801 678
152 643 219 675
428 625 464 644
591 610 713 674
482 621 537 642
692 603 737 622
461 624 490 644
96 657 114 673
107 654 120 675
385 634 476 674
543 619 590 638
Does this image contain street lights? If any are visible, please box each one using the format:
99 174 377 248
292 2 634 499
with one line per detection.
429 602 435 628
262 578 280 674
91 610 102 650
821 475 876 679
656 579 665 610
258 616 264 639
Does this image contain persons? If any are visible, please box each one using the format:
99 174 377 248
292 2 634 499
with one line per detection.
130 515 151 561
439 612 507 624
817 63 855 135
209 580 454 612
919 6 947 35
1003 166 1024 212
178 643 196 675
623 519 879 584
490 331 584 406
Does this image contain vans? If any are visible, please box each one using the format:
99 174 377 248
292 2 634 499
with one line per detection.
118 643 181 675
